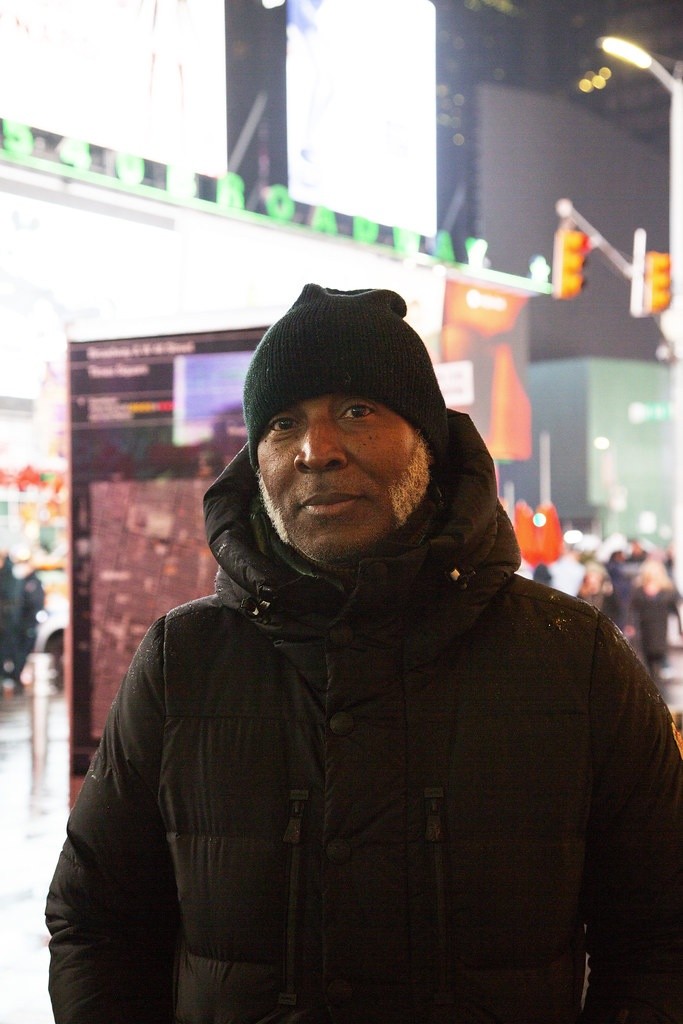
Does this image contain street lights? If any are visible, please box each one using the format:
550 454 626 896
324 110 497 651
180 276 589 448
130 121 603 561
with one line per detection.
598 36 681 331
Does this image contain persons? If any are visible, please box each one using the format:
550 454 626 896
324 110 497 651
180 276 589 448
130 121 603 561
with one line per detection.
0 552 68 700
531 530 683 684
46 284 683 1023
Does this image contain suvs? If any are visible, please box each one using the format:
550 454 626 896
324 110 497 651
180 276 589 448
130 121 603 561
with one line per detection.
31 551 68 689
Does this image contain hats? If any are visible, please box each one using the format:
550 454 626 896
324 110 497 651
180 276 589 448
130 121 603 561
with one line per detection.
244 283 450 474
